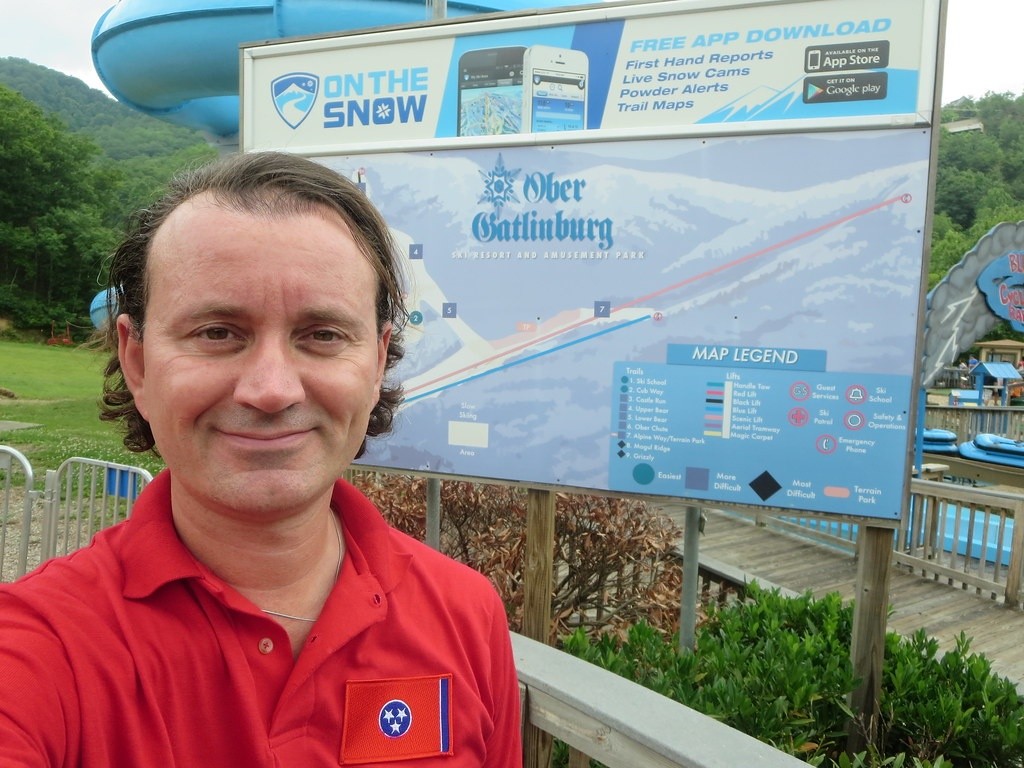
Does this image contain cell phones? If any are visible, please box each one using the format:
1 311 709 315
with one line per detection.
457 45 589 137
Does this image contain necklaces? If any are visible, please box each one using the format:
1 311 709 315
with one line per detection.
259 508 343 623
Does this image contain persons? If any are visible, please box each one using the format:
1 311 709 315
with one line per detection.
1018 355 1024 379
1009 380 1022 399
960 359 968 389
969 354 979 384
0 155 520 768
